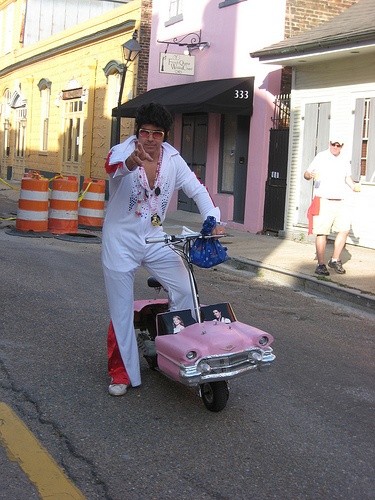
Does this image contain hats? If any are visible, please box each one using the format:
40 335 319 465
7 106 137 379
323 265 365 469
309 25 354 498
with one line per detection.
329 134 344 145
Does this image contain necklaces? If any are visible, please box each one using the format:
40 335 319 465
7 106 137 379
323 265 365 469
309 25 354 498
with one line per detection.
142 147 164 213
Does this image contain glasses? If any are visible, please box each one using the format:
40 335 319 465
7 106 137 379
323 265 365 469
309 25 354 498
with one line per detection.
139 128 165 140
331 143 341 148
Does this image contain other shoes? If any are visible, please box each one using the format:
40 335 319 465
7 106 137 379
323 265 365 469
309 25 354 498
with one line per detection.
108 378 128 396
315 264 329 276
328 257 345 274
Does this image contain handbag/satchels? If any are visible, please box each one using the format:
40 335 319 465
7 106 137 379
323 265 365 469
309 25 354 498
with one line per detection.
188 216 230 268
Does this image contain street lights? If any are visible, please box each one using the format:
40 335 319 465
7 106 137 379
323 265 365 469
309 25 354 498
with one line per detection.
116 27 141 145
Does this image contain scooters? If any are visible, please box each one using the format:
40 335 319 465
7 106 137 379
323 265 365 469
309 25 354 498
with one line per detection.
132 234 277 413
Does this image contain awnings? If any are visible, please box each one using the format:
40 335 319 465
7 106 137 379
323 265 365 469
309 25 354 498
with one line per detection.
111 77 254 118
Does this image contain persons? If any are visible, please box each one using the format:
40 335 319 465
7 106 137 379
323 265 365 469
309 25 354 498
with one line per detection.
212 306 231 323
100 101 226 396
172 315 185 333
304 133 362 276
